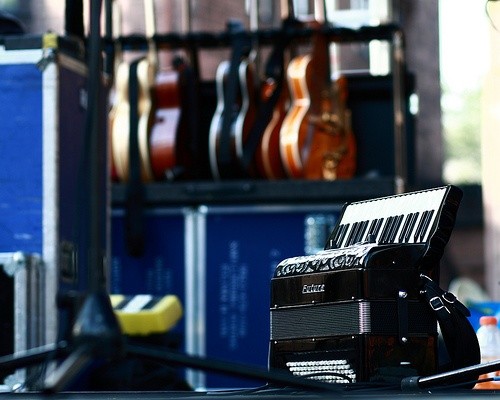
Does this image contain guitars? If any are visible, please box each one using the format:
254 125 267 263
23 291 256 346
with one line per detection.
280 0 354 180
102 0 289 178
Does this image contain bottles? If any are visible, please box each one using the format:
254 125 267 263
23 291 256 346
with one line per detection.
472 316 500 389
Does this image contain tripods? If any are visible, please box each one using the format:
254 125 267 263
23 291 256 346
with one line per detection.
0 0 350 400
